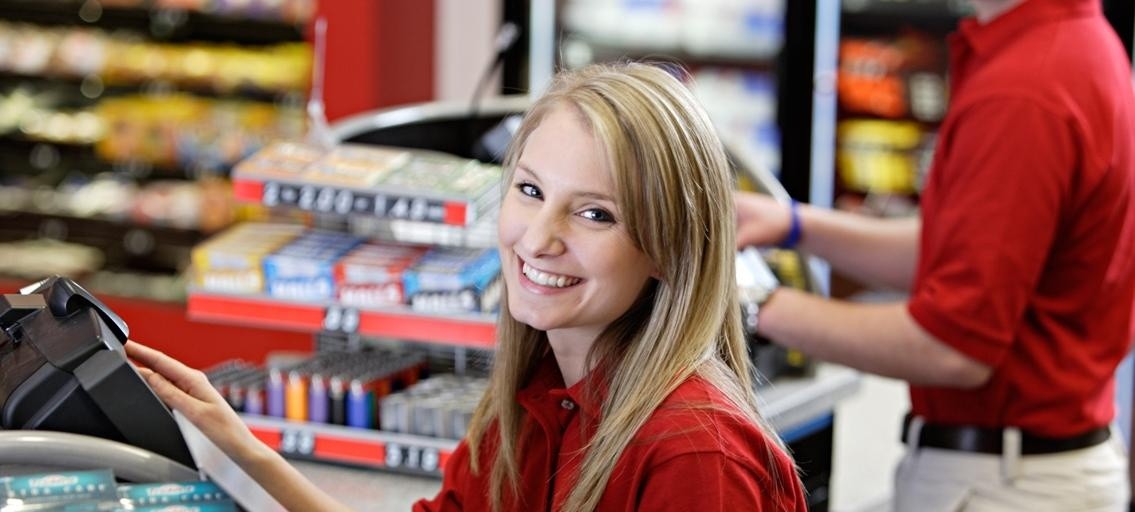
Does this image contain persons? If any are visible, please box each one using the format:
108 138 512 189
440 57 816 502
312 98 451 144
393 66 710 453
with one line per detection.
728 0 1134 511
121 50 808 512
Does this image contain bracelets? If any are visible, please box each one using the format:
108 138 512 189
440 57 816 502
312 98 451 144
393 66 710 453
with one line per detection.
775 198 803 250
746 283 777 338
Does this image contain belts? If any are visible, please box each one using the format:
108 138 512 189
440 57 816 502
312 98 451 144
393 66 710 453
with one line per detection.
903 413 1110 455
